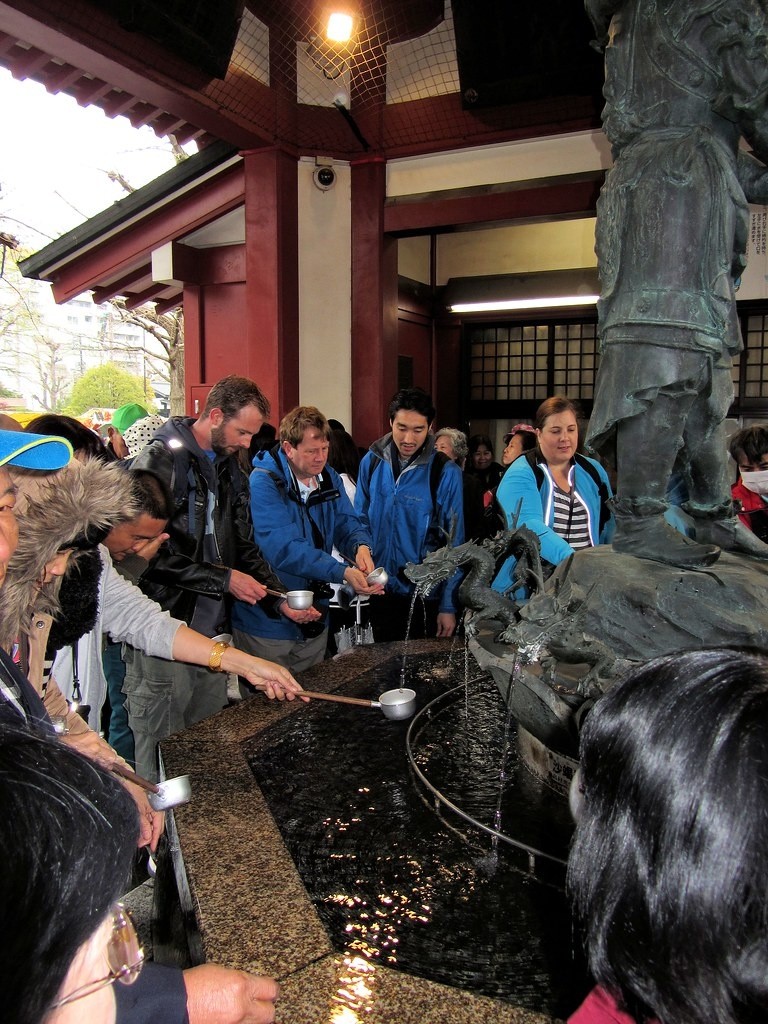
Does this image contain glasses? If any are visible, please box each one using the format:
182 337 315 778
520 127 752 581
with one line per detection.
47 902 150 1010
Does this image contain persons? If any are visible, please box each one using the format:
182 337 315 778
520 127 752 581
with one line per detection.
0 720 144 1024
0 375 768 852
585 1 768 570
566 650 768 1023
0 431 278 1024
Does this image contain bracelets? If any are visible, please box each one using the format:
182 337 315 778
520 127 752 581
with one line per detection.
210 641 229 672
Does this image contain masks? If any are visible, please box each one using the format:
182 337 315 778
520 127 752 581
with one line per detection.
739 467 768 494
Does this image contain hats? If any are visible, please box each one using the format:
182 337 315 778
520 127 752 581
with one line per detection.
112 403 149 435
1 429 71 471
503 424 535 443
123 415 167 461
